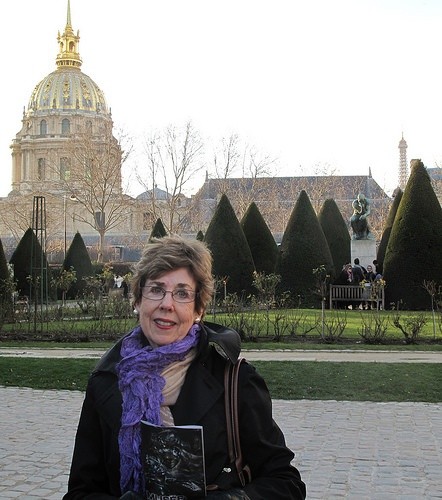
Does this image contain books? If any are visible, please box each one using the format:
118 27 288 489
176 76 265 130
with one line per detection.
140 420 206 500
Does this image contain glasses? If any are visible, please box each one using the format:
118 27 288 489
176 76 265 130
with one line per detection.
139 285 199 303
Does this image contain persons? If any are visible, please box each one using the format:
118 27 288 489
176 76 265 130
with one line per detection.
19 289 24 301
116 275 128 301
334 258 383 309
62 236 306 500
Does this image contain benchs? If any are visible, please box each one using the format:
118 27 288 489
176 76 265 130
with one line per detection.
329 284 385 309
